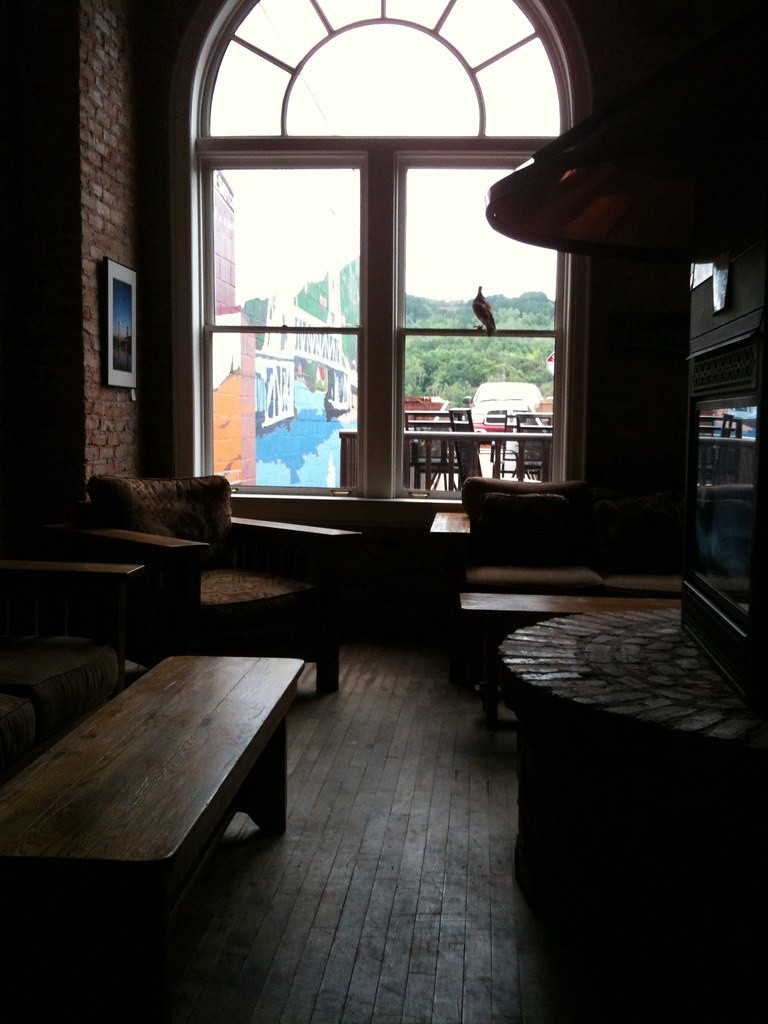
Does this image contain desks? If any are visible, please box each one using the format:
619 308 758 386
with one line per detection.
459 592 680 730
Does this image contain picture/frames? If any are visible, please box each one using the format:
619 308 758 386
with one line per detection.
103 256 140 390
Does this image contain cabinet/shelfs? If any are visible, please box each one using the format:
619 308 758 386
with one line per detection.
679 224 767 708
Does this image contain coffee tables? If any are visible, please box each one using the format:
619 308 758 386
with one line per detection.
0 657 306 1024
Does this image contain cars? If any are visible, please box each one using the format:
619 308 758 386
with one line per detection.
462 382 544 432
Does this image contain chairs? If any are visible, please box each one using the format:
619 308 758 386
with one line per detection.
403 411 553 491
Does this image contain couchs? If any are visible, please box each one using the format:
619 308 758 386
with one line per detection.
429 481 607 690
589 482 683 598
1 555 146 786
67 474 364 694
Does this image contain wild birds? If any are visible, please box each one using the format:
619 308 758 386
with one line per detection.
473 286 496 337
